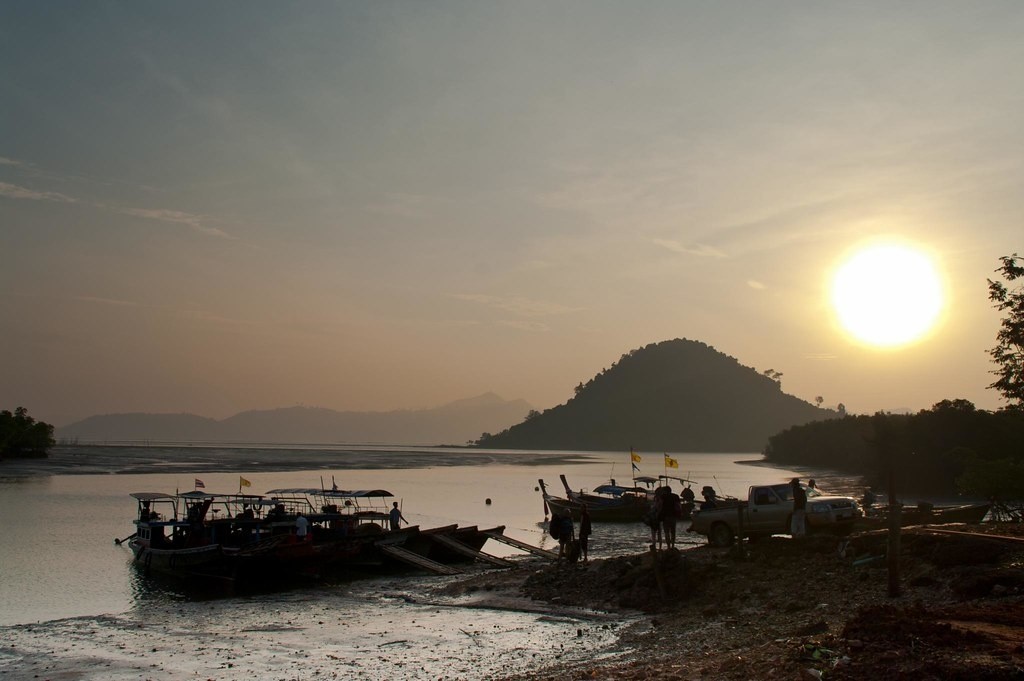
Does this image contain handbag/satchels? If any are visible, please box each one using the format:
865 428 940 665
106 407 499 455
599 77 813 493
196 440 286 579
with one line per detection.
674 502 681 518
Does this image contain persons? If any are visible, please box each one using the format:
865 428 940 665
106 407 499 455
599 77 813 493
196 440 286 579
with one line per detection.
650 486 679 550
791 478 807 537
579 511 591 561
390 502 408 530
804 480 820 496
558 508 575 557
296 512 309 536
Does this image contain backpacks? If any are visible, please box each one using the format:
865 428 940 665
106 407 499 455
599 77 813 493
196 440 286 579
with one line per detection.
559 517 572 535
550 513 559 539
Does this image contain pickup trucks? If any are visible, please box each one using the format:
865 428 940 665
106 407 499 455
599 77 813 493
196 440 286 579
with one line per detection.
692 483 864 547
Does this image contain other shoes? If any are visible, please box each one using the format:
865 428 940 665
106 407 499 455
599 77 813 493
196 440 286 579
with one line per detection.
655 548 675 552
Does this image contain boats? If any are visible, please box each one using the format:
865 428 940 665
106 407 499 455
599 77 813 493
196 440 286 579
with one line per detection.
872 501 990 526
539 479 644 522
127 493 222 578
178 487 505 589
560 475 697 516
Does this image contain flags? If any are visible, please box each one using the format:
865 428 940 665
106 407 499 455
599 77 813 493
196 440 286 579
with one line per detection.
195 479 205 488
632 452 641 462
665 454 678 468
240 476 251 487
